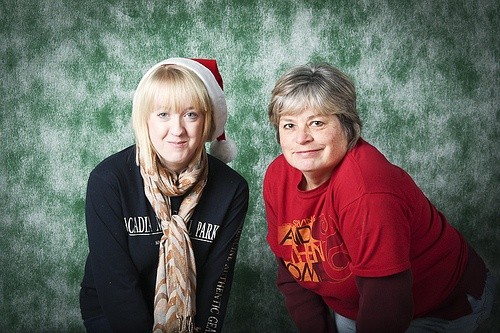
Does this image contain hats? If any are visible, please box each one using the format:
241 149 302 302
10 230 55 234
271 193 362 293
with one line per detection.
134 59 236 162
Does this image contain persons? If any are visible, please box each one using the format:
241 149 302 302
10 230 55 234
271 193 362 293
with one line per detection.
263 64 492 333
79 58 249 333
